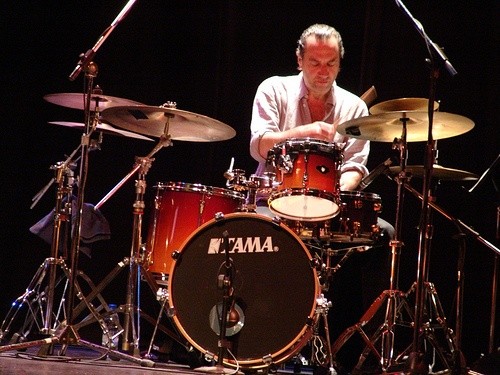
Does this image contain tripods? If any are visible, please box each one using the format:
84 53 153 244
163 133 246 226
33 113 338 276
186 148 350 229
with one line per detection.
0 0 500 375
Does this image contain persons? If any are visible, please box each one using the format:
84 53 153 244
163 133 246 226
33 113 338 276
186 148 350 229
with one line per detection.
248 22 399 371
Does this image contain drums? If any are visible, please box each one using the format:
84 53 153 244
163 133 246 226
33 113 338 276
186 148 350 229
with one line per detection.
142 181 246 279
268 138 342 221
282 190 381 243
167 211 323 369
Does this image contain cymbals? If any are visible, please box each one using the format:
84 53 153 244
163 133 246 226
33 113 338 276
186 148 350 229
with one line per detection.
389 165 472 178
369 98 440 115
43 93 146 111
336 111 475 142
100 106 237 142
49 121 155 142
